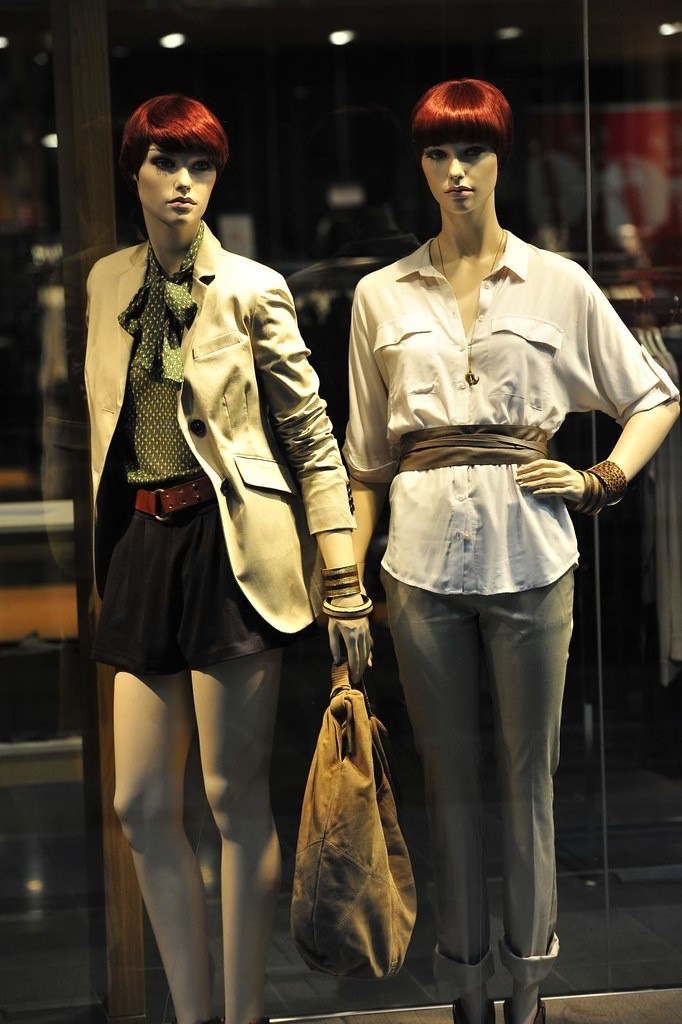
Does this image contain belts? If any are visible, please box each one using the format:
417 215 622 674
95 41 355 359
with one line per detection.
133 477 216 522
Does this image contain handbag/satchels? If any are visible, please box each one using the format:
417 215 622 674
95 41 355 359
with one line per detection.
291 663 417 980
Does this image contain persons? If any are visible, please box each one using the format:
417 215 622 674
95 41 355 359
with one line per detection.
77 92 375 1023
309 77 682 1024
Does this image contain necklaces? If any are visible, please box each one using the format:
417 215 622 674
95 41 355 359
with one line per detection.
435 228 505 386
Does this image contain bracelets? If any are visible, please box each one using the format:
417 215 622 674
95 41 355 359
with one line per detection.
321 594 374 618
584 460 627 505
566 468 609 517
319 563 361 599
